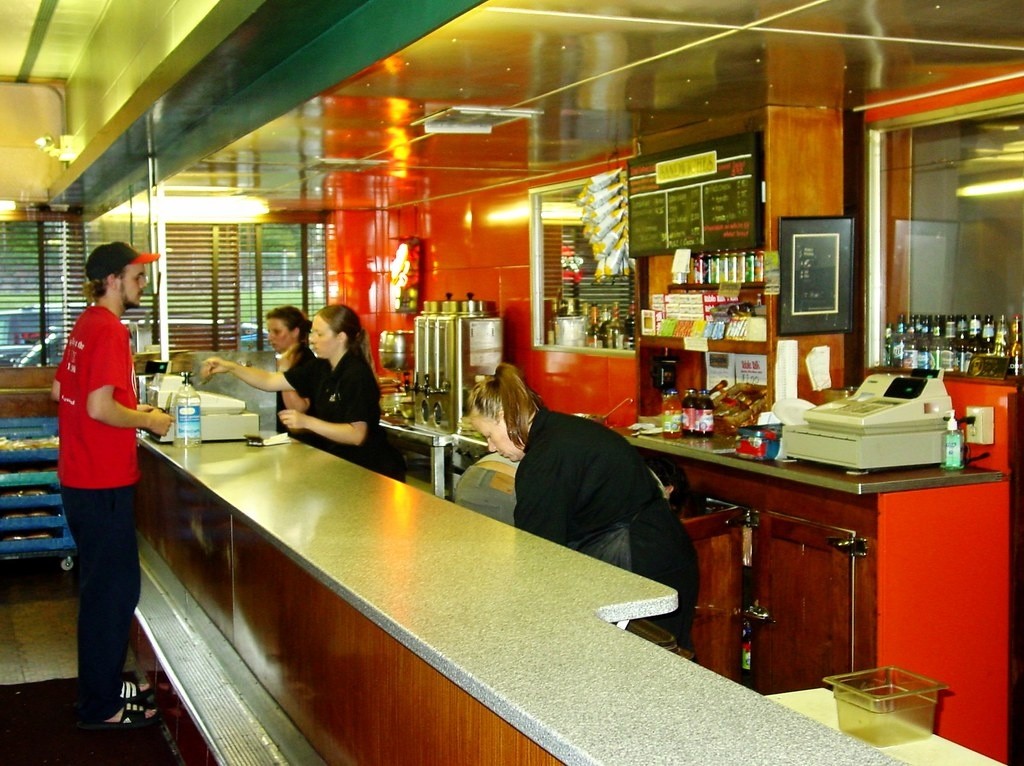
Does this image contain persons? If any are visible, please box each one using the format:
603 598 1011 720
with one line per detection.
198 303 389 479
50 240 178 730
468 363 699 666
265 305 318 435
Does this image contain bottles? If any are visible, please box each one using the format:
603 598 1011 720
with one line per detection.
981 314 995 355
682 390 696 435
930 314 944 372
891 314 906 368
994 315 1009 357
953 314 968 374
941 315 956 374
741 627 751 675
546 299 568 345
662 389 682 439
883 323 893 367
582 301 635 351
1006 314 1024 377
965 315 982 374
904 315 919 369
696 390 714 437
918 314 932 369
176 371 202 448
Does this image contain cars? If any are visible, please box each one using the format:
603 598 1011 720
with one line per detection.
0 305 272 366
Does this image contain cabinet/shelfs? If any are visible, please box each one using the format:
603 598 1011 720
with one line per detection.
632 447 1012 766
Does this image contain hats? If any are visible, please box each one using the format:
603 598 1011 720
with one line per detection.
85 241 161 281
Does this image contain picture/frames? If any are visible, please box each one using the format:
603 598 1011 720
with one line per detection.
776 216 855 338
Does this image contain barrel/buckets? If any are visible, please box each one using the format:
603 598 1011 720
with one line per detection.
554 316 584 346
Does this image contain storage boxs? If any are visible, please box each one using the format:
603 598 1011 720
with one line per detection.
823 664 949 747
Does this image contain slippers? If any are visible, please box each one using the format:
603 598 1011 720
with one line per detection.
77 702 160 728
120 680 154 702
